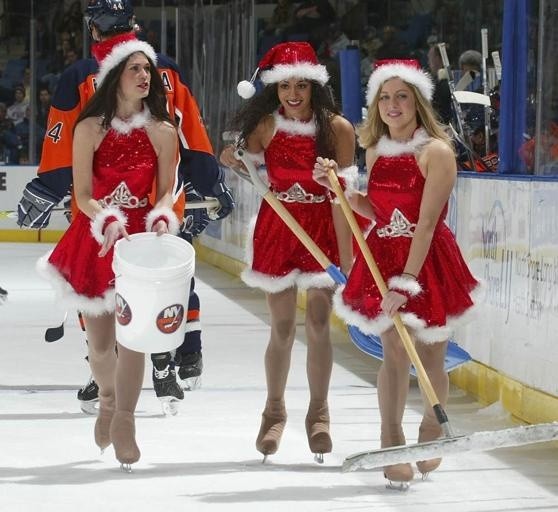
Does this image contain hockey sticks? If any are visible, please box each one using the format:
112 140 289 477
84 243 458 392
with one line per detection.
452 91 491 173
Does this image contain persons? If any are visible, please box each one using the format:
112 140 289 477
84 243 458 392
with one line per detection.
310 56 491 489
33 30 182 471
3 3 80 161
176 245 205 394
218 40 360 462
14 3 238 407
258 1 555 98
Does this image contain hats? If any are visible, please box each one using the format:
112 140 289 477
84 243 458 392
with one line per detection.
15 83 25 97
91 34 158 86
236 40 331 98
366 59 435 108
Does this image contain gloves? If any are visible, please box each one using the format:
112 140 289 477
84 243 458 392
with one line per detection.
206 183 236 220
179 184 210 237
17 182 62 229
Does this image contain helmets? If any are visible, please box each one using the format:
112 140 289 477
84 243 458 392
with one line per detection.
84 1 134 37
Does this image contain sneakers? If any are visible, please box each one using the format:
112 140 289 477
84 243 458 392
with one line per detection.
111 411 140 464
415 414 442 472
153 369 184 400
379 423 414 481
179 350 204 377
79 379 98 401
305 407 332 452
94 395 115 447
256 404 287 453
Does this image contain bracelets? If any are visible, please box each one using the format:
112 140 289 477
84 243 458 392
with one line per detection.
145 208 180 235
401 270 418 280
330 176 355 205
88 208 127 240
389 272 422 298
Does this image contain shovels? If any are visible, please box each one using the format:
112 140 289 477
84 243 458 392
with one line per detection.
230 148 471 379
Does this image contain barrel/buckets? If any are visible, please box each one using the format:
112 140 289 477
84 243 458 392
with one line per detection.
108 231 197 355
